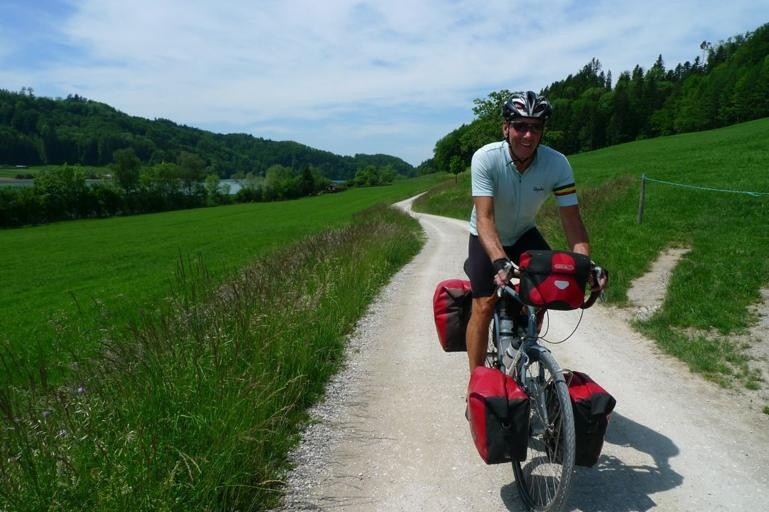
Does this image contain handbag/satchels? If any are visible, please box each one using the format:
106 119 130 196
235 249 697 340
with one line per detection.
505 283 546 341
518 250 590 309
432 279 474 352
541 371 616 469
463 366 530 465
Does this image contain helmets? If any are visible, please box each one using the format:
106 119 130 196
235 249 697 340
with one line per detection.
502 90 553 120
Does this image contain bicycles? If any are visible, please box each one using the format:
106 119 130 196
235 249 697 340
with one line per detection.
485 261 599 512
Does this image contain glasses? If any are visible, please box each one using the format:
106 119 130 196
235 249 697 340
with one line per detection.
508 121 543 133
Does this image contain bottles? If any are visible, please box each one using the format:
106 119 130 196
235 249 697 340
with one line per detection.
498 316 521 370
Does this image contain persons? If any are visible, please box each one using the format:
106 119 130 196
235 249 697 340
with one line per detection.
460 90 609 428
